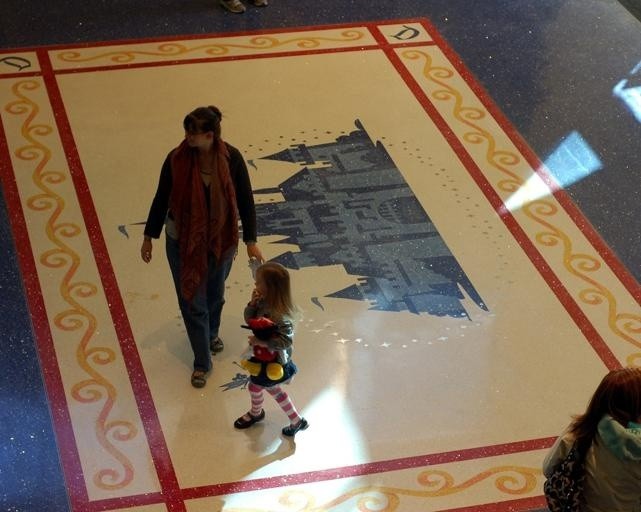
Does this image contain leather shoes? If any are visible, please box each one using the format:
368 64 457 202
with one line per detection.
234 409 266 429
283 418 311 436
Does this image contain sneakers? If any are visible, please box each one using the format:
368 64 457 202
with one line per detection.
191 367 211 387
210 335 225 352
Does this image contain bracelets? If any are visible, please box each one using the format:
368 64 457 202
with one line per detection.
142 236 152 242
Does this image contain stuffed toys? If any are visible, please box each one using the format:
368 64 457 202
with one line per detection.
238 315 290 383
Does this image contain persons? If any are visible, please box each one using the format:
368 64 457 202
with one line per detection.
539 366 641 512
234 259 310 439
219 0 268 14
141 105 266 390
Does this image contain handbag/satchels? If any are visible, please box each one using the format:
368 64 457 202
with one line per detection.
541 448 592 511
